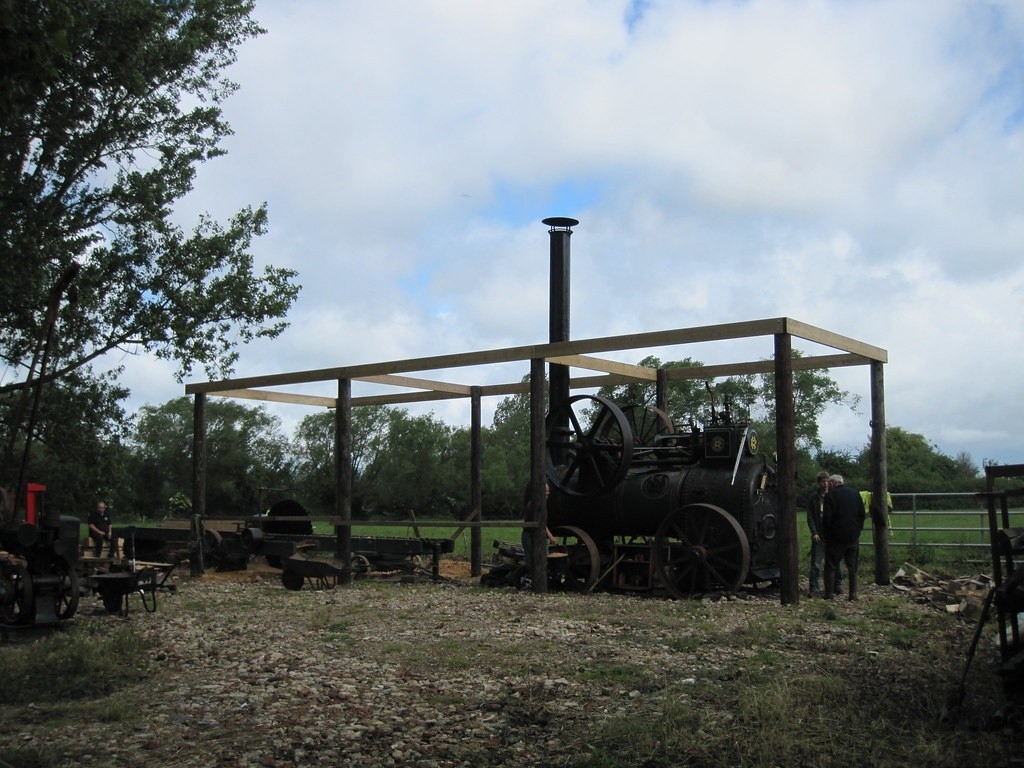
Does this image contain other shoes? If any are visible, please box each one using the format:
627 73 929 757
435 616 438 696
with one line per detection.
833 585 846 594
808 588 820 598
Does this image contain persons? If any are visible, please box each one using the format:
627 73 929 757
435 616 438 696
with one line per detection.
804 470 866 603
88 500 118 560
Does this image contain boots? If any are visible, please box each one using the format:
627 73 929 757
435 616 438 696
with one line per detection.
822 571 835 599
849 571 859 600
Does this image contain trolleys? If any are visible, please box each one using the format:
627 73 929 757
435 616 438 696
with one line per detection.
88 560 183 617
282 551 374 590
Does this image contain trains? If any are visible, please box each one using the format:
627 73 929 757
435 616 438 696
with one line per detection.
523 380 781 600
